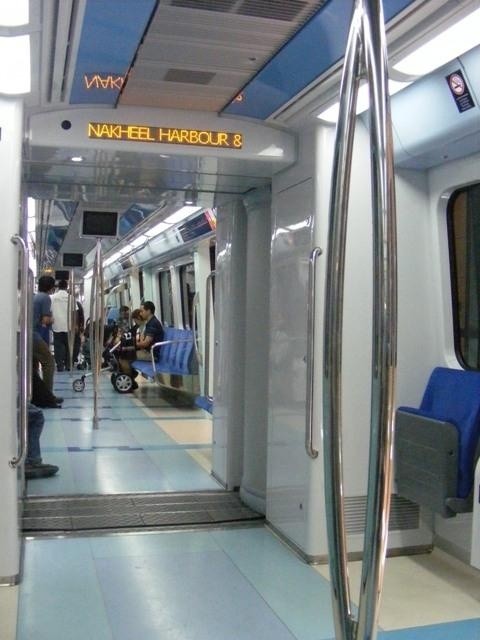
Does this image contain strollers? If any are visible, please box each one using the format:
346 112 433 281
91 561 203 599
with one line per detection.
72 318 139 394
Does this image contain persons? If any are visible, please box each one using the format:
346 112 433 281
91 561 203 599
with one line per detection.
16 276 167 409
17 398 59 480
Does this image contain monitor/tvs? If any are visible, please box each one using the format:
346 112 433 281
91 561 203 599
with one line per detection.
79 207 120 239
55 269 70 281
61 252 84 269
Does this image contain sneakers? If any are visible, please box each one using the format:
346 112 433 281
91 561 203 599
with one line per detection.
25 462 59 478
57 363 71 372
132 371 138 389
49 395 64 408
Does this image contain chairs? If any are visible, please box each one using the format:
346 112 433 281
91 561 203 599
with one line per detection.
130 327 200 395
393 366 480 515
195 395 213 414
